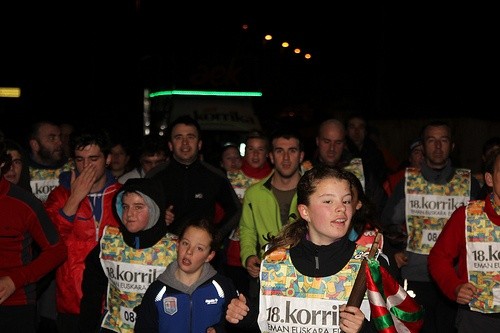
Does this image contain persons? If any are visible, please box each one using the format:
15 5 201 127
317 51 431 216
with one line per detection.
0 114 500 333
